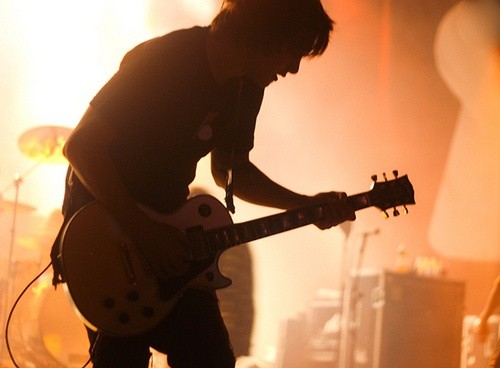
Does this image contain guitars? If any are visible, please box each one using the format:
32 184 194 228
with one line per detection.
56 169 416 339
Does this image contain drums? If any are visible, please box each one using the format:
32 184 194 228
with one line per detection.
16 275 93 368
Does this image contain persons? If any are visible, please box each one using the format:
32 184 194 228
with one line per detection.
50 0 355 368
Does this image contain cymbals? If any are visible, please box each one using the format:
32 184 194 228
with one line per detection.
16 125 74 164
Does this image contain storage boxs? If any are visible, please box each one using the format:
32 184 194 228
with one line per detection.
340 270 464 368
